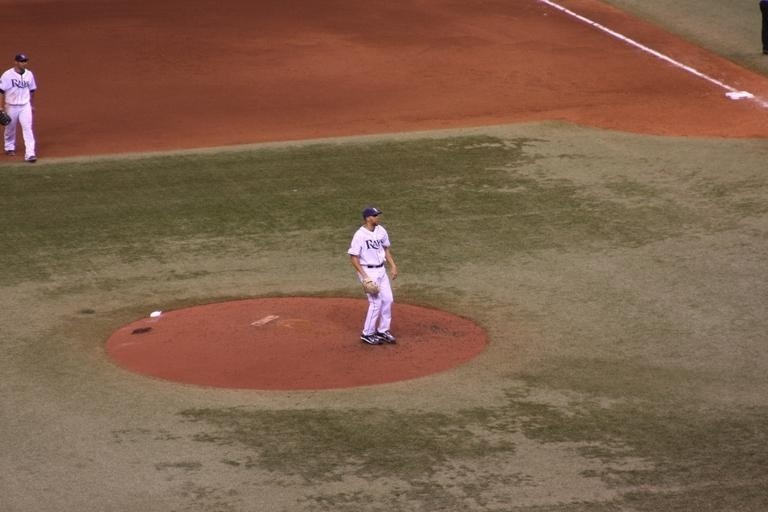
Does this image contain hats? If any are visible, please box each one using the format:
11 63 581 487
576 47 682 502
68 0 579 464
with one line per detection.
364 208 381 216
15 54 28 62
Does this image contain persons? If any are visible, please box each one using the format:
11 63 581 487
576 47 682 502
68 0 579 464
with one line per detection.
759 0 768 54
0 54 38 162
347 207 400 345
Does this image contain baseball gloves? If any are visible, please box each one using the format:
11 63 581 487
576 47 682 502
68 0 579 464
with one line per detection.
0 110 12 126
364 278 380 297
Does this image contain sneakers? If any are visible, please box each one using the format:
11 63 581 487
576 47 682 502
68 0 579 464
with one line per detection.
377 330 396 343
28 156 37 160
8 150 15 156
360 335 384 344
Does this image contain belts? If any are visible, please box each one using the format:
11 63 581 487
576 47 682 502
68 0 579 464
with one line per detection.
368 263 384 268
